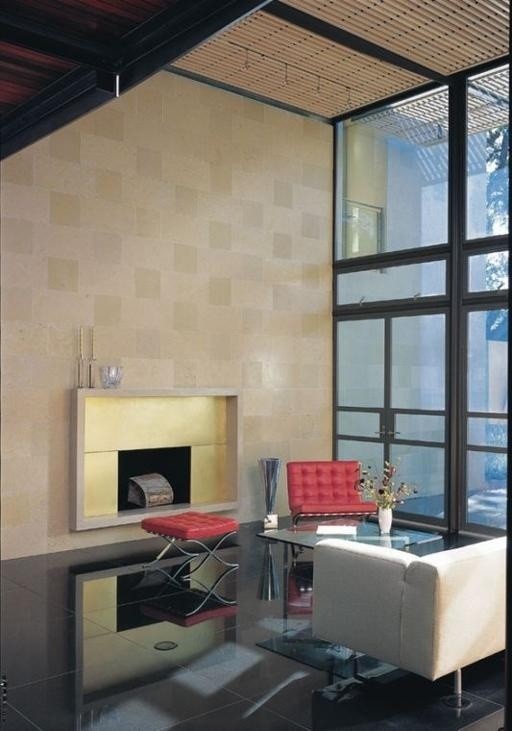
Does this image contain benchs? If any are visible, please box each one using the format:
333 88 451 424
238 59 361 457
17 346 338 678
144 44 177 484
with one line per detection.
284 546 315 617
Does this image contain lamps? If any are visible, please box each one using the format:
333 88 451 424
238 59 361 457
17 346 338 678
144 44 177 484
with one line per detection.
244 47 353 108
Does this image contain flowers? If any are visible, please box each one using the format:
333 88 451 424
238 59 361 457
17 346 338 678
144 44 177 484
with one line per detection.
352 456 418 510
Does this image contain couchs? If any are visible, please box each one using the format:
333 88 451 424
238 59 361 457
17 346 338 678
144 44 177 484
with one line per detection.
304 537 509 699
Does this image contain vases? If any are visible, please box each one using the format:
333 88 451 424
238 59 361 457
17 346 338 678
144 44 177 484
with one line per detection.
377 504 393 534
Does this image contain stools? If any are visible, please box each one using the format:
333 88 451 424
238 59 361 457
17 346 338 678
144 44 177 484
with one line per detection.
286 460 379 528
137 566 240 628
137 511 244 579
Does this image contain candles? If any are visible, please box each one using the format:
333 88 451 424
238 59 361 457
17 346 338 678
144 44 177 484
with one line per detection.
91 326 95 359
79 326 85 359
